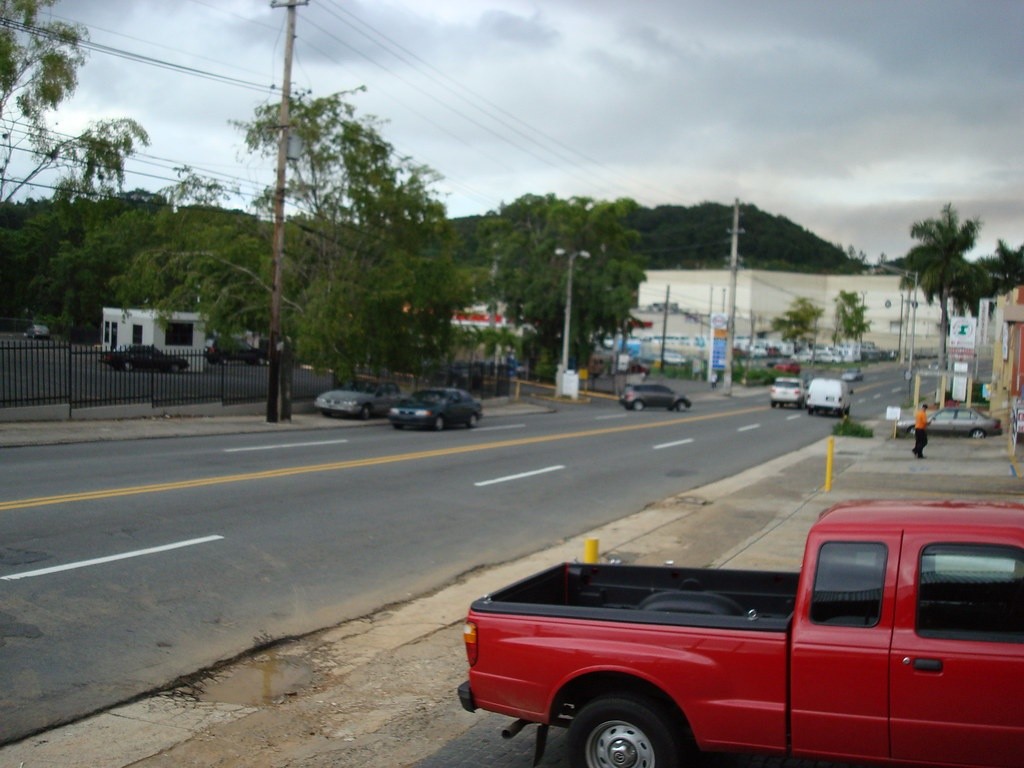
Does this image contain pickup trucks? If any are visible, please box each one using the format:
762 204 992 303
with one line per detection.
457 495 1024 768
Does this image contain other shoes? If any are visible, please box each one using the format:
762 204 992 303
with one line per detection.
912 449 917 455
918 455 926 458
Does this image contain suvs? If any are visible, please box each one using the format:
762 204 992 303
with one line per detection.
617 384 692 412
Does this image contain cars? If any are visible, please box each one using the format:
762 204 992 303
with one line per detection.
896 405 1004 441
840 366 864 382
387 387 484 432
770 375 806 408
97 343 190 374
26 324 51 340
313 378 403 421
203 338 267 366
775 346 844 374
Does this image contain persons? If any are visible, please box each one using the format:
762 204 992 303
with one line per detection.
911 404 931 458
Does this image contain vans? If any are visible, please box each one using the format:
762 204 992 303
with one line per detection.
806 376 855 418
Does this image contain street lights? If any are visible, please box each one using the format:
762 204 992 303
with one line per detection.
555 248 591 372
